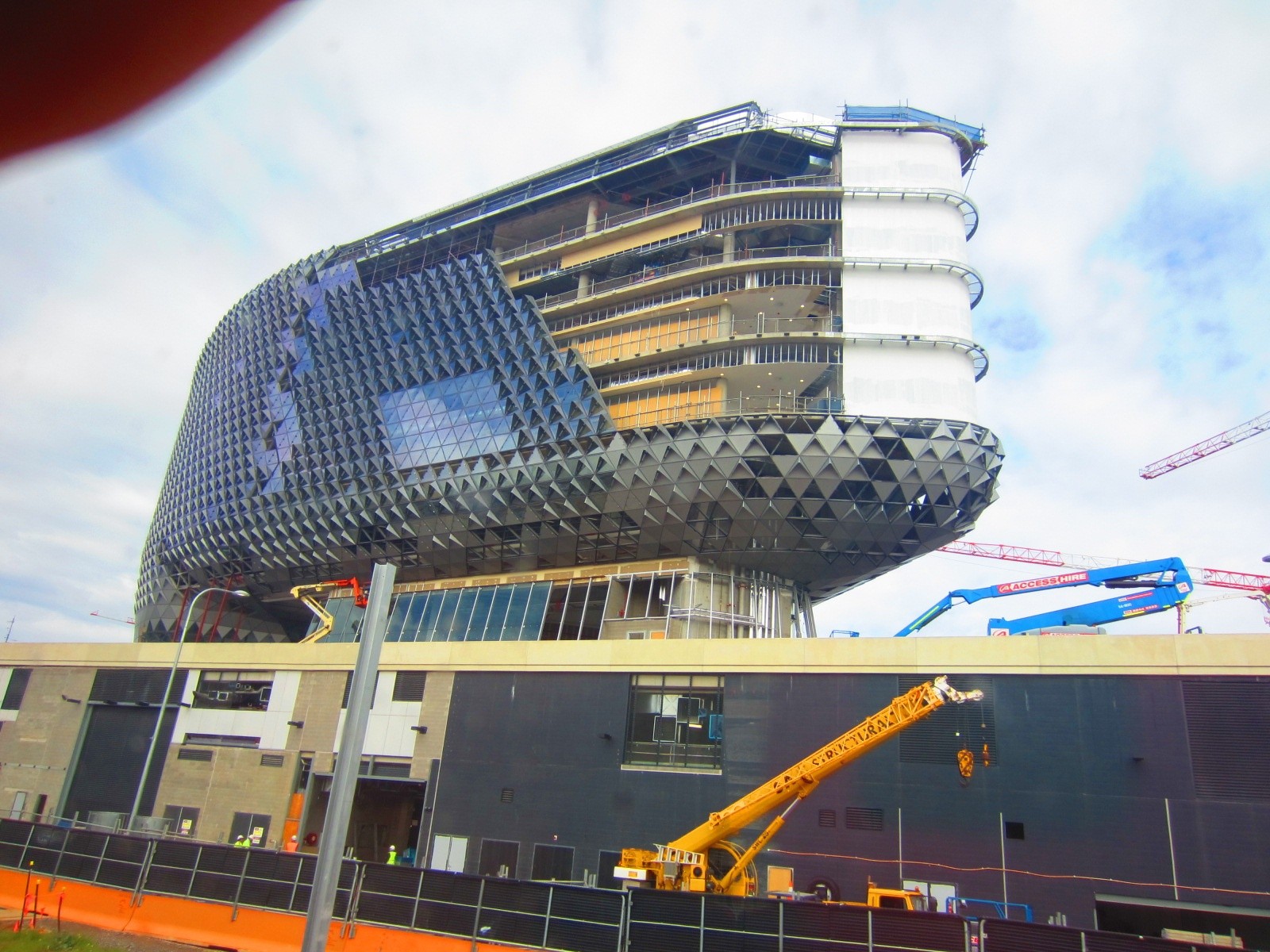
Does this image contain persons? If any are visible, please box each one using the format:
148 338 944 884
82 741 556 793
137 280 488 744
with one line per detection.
385 845 399 865
235 835 254 849
286 835 298 851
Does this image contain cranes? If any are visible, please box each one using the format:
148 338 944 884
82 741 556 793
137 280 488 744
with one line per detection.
1139 411 1270 479
90 611 135 624
941 539 1270 623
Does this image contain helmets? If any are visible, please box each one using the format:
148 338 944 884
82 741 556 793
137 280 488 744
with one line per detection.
291 835 297 840
237 835 244 840
249 834 254 839
390 846 395 851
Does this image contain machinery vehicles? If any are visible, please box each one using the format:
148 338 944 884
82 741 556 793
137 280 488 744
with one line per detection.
829 555 1194 634
619 673 985 911
290 577 371 643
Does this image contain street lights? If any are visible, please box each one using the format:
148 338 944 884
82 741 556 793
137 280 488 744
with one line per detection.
125 588 252 835
